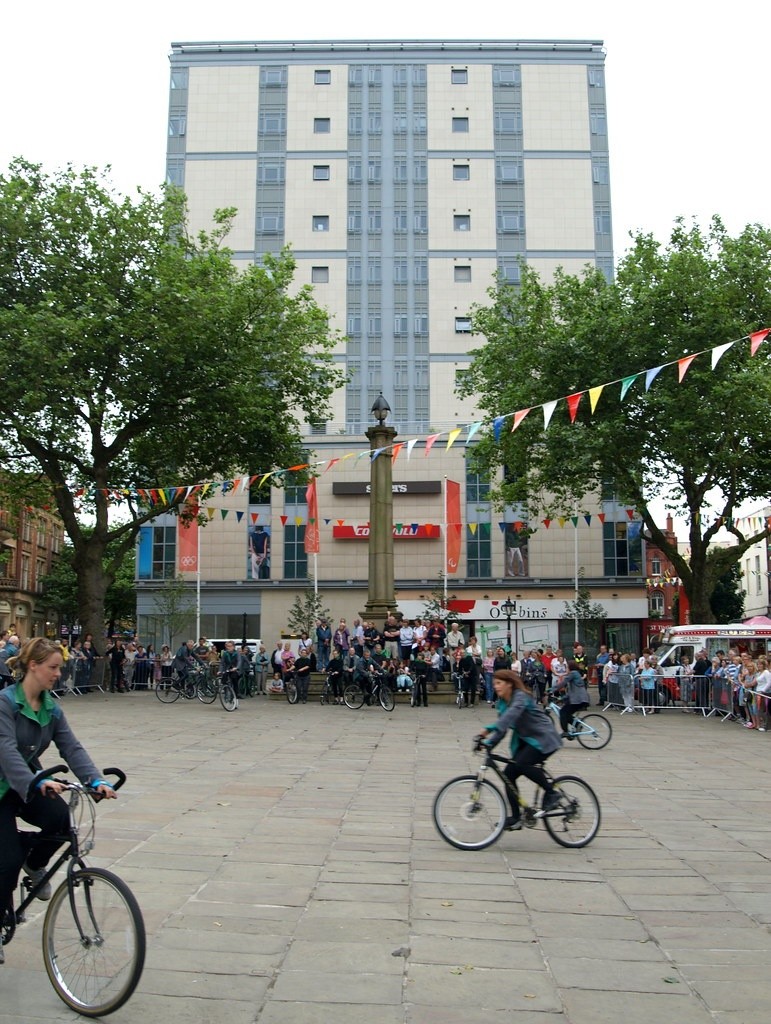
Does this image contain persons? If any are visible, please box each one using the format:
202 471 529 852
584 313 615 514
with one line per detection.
298 632 312 656
106 638 254 703
255 646 269 695
0 623 103 698
594 645 771 732
507 525 526 576
305 646 317 672
0 638 117 964
312 611 588 707
544 660 590 741
476 670 563 830
270 641 310 704
250 526 268 579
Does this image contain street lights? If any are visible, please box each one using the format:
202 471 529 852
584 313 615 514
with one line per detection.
501 596 514 655
358 388 404 641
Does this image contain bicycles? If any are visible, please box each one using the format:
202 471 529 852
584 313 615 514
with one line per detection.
407 673 424 707
1 765 146 1017
343 671 396 711
155 663 257 712
432 740 602 851
285 670 307 704
542 692 613 750
318 670 335 706
452 672 468 708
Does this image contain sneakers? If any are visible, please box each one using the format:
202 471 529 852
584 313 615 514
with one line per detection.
22 859 51 901
0 936 5 963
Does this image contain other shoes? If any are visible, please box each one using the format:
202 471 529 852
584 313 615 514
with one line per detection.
333 701 339 705
340 700 346 705
423 701 428 707
543 792 562 811
461 703 468 707
172 683 181 690
467 704 474 708
110 688 115 693
683 708 768 731
302 699 307 704
560 732 571 738
416 699 421 706
596 702 604 706
237 694 245 699
366 699 371 706
647 710 655 714
502 816 522 830
117 689 124 693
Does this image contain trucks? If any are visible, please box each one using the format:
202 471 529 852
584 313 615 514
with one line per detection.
635 616 771 705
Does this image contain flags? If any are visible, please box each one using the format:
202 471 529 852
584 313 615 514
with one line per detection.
447 480 461 573
178 500 197 571
304 477 319 553
